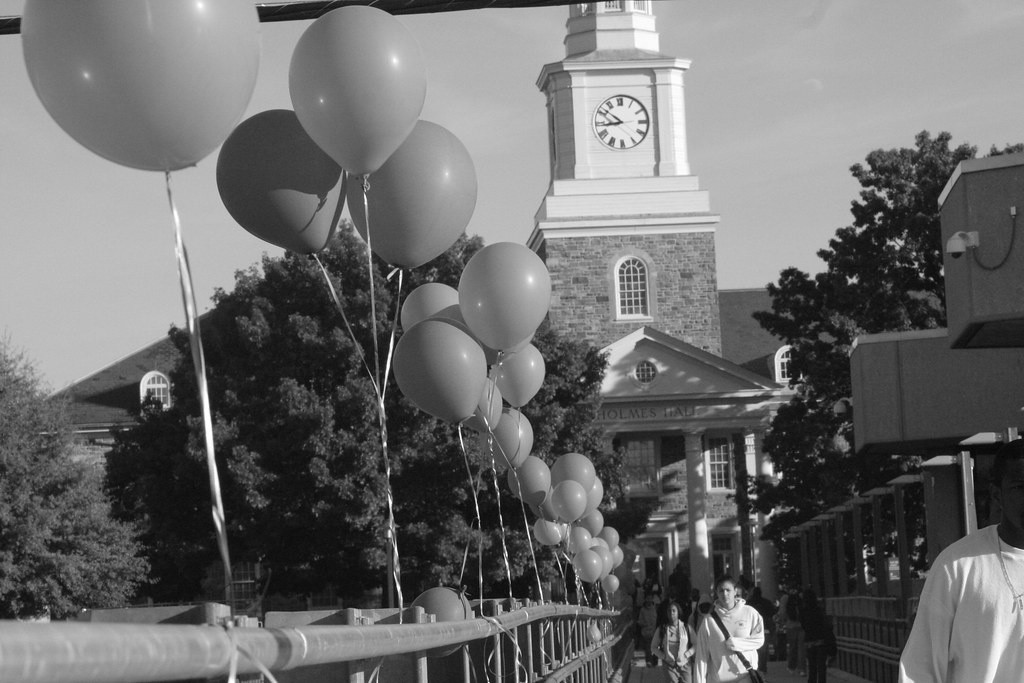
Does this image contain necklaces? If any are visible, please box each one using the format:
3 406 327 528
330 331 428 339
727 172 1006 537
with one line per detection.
998 526 1024 610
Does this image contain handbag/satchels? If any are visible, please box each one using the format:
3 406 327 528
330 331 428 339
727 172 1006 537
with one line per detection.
822 621 838 655
749 669 766 683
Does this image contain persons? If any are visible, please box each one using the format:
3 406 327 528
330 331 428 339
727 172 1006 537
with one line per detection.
634 562 837 683
898 440 1024 683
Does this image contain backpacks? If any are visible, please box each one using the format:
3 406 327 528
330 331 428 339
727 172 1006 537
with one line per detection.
786 591 804 622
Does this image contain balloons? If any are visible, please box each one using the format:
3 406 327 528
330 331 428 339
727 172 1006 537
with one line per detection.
19 0 261 171
217 5 624 653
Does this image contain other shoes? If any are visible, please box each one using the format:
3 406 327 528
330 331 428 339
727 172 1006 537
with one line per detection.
787 667 795 674
647 662 652 667
801 672 807 676
